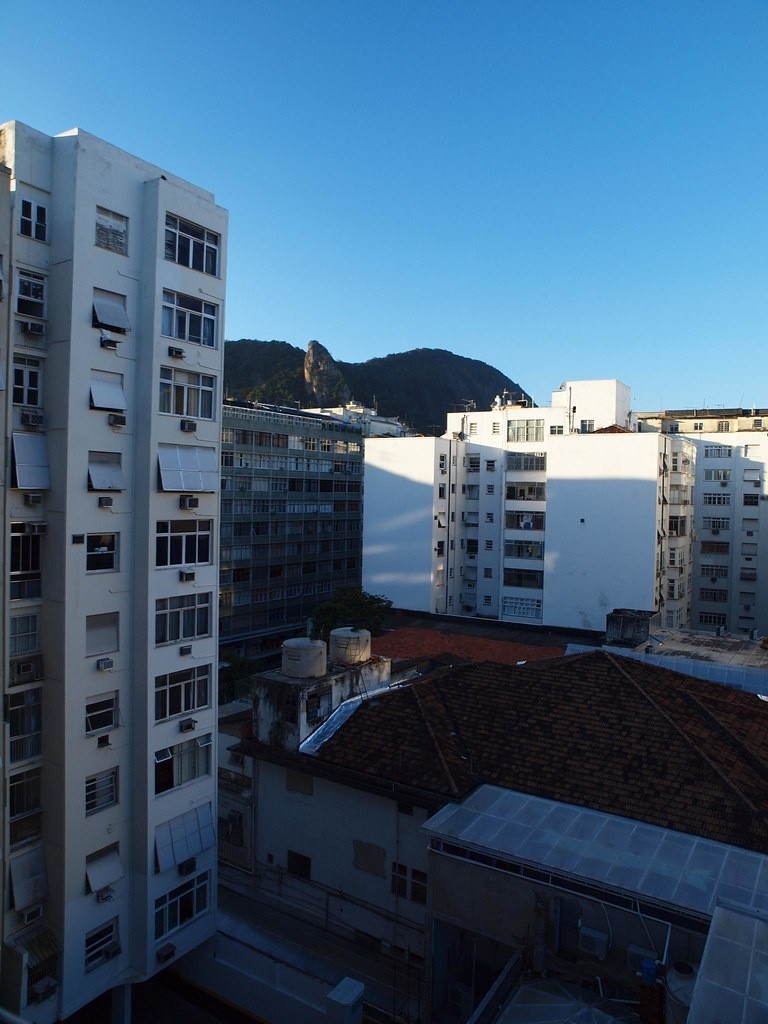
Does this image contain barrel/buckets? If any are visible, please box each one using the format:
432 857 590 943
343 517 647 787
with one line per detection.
641 959 658 984
749 629 758 639
645 645 655 653
715 625 724 636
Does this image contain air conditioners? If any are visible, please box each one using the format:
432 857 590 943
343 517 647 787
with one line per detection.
627 944 658 970
97 659 114 670
577 927 609 960
108 413 127 428
21 904 43 925
25 413 44 426
35 983 56 1003
181 497 199 509
180 570 196 583
25 322 45 336
17 661 33 676
99 497 113 508
180 646 191 656
24 492 42 504
181 421 197 432
181 722 195 733
104 943 120 961
179 860 197 877
